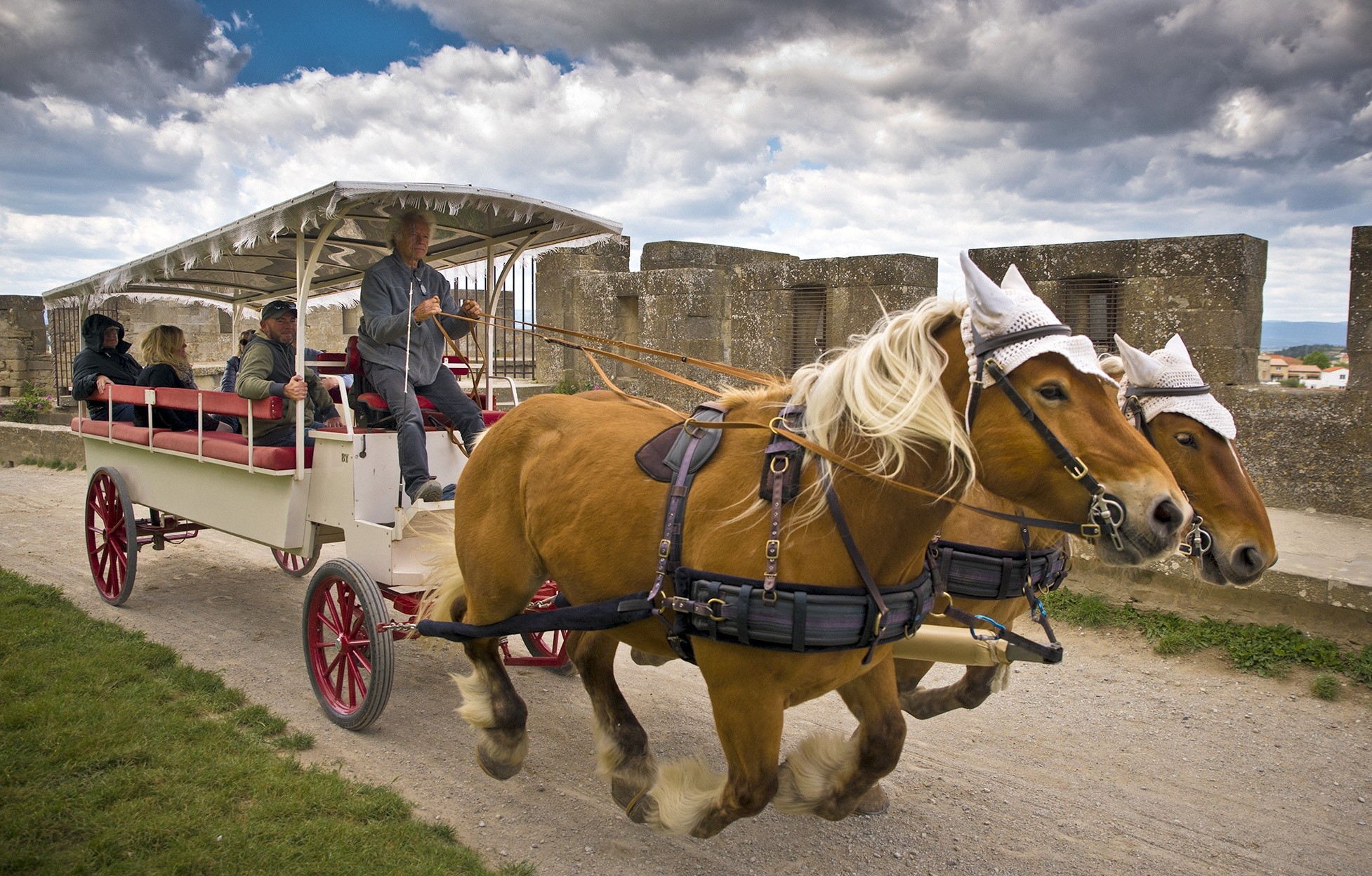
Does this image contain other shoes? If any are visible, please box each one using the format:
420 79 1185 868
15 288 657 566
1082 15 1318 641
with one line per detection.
414 481 442 501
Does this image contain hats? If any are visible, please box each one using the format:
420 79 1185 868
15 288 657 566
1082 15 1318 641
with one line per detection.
262 299 297 322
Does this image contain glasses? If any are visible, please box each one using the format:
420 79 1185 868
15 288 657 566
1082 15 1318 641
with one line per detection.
239 339 248 345
413 270 428 296
261 301 297 320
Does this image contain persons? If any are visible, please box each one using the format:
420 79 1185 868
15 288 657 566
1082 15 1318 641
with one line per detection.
356 203 488 504
72 299 354 449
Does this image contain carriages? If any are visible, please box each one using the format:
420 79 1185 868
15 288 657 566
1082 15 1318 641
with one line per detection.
66 179 1282 845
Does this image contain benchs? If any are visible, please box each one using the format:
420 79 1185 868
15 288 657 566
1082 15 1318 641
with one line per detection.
70 376 315 470
311 347 497 427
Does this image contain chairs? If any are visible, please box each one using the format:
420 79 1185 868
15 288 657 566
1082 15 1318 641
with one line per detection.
344 335 438 424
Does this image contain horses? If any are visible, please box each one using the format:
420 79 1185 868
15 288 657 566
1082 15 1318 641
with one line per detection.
418 250 1279 839
